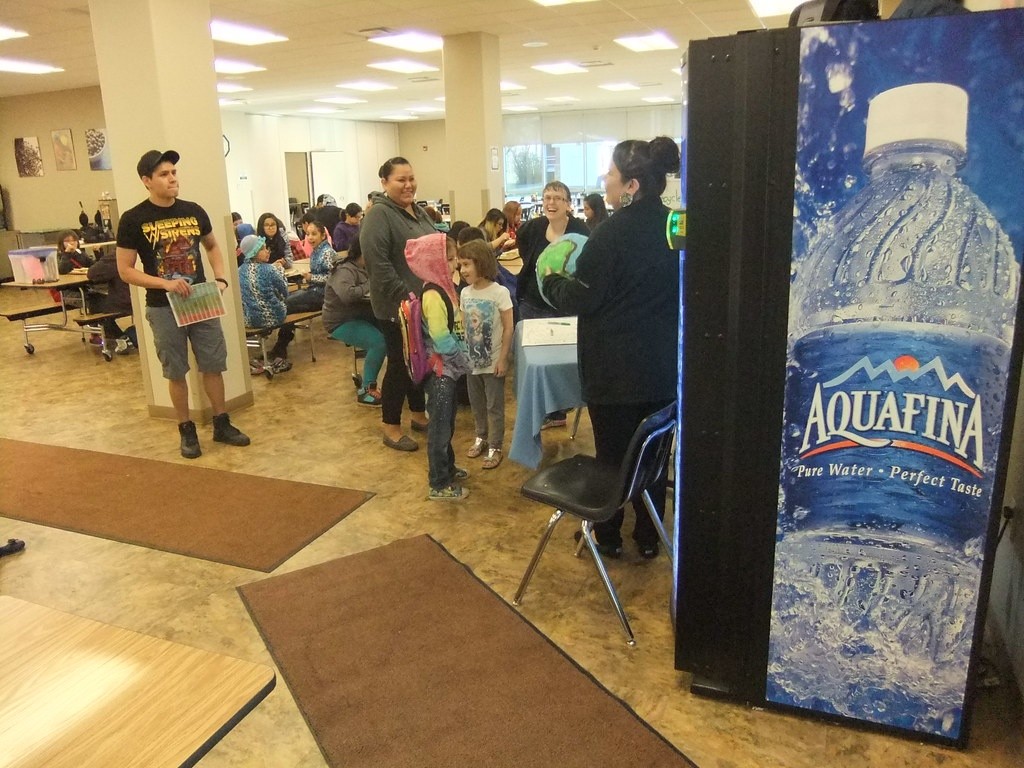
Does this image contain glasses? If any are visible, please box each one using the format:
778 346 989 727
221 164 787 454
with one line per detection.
355 215 363 220
263 224 278 230
543 195 570 203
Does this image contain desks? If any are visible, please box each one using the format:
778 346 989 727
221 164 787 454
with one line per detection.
507 317 584 470
441 215 451 228
30 239 117 249
285 249 349 299
1 275 112 332
518 199 614 223
1 595 278 768
363 249 524 300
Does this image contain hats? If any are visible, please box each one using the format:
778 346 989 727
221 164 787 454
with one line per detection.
240 235 266 258
136 149 180 180
257 213 280 239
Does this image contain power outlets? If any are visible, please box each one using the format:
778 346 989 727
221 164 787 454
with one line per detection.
1004 497 1019 541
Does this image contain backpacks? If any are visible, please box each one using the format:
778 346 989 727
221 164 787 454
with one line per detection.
398 283 453 382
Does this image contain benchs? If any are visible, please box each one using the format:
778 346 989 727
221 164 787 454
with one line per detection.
288 284 309 293
244 310 323 380
73 312 133 362
0 293 77 354
327 334 368 388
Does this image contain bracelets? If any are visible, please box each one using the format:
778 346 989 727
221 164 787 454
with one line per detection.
215 278 229 288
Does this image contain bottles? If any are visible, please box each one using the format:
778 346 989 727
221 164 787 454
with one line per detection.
767 82 1020 738
102 191 111 200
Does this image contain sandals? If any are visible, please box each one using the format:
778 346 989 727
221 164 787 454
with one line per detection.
482 449 503 468
466 438 487 458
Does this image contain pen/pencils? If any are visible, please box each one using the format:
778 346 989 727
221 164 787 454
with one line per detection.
548 322 570 325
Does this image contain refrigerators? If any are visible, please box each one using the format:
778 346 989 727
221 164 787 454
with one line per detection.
673 9 1024 748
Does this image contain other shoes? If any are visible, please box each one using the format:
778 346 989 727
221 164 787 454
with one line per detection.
383 433 419 452
575 530 622 559
638 542 659 558
411 419 429 432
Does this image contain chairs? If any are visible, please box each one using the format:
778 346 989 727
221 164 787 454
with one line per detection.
514 402 679 647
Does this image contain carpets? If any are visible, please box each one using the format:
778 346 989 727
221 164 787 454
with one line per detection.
0 438 377 573
234 534 697 768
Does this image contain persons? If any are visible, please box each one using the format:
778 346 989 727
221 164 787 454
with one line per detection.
257 212 293 269
423 205 449 232
542 136 680 558
277 219 306 260
517 181 591 320
502 201 523 250
300 213 331 257
333 203 363 252
479 209 515 258
85 248 133 353
116 150 250 458
359 157 437 451
447 222 514 468
404 233 473 499
238 235 293 373
266 220 338 360
321 232 386 407
232 211 255 267
302 194 343 236
584 194 608 231
57 229 105 346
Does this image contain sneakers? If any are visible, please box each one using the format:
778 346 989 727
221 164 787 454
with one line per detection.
429 486 470 500
114 337 134 355
457 468 466 479
90 336 103 346
357 382 382 408
273 360 292 373
213 413 250 446
250 357 267 374
542 412 567 429
178 421 201 458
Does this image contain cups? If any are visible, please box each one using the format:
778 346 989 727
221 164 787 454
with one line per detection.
88 132 110 170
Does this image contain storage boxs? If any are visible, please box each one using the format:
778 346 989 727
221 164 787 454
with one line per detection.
8 248 59 284
